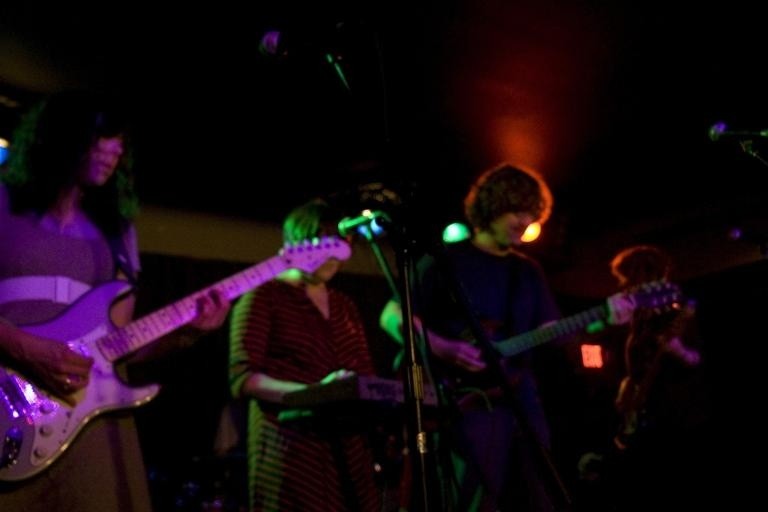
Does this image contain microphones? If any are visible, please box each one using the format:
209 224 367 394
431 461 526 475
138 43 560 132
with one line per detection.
336 210 382 238
707 127 768 142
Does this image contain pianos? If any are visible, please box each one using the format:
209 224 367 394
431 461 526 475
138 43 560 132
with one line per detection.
274 366 450 424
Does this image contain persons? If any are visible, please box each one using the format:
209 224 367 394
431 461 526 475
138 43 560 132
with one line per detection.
0 87 231 512
228 195 389 512
375 159 638 512
607 243 716 511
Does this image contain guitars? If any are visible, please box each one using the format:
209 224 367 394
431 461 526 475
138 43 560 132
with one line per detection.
1 235 351 483
394 278 678 410
610 302 697 449
